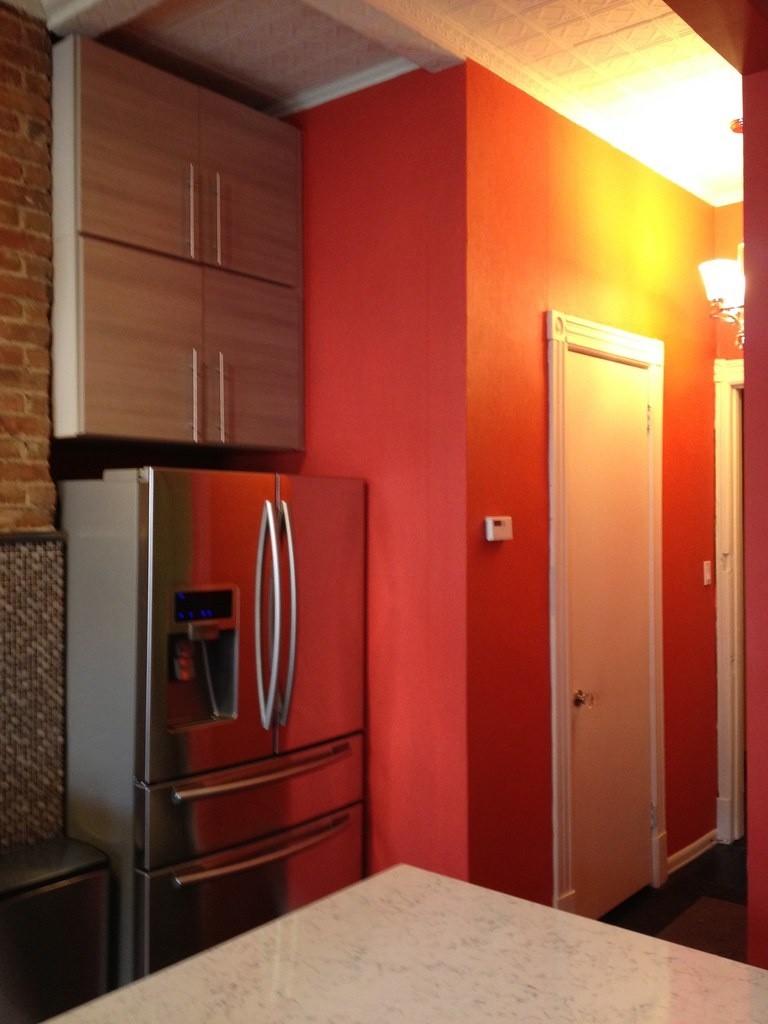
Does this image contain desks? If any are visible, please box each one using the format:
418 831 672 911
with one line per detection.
42 863 768 1024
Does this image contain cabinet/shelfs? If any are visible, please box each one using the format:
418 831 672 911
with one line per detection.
48 34 306 451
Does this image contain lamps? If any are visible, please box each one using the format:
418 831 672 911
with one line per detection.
702 238 745 343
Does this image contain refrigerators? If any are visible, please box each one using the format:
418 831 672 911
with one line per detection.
54 465 368 991
